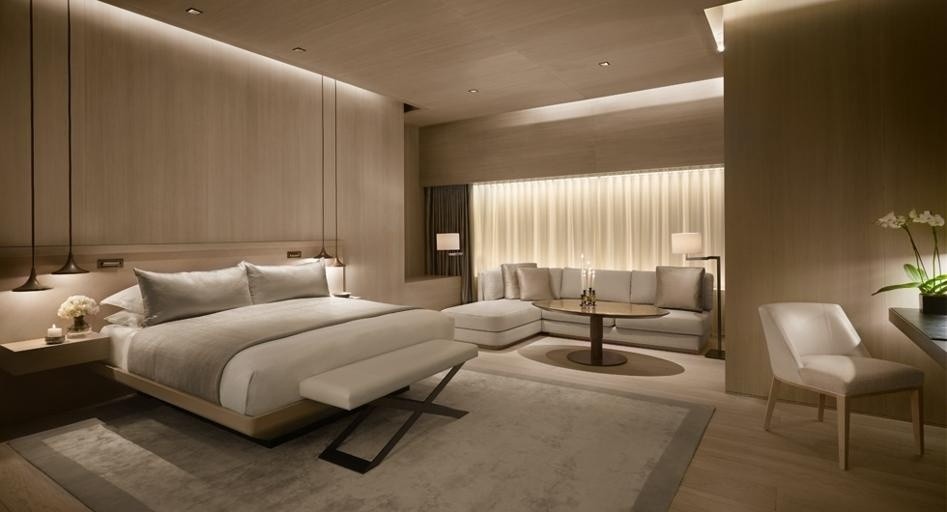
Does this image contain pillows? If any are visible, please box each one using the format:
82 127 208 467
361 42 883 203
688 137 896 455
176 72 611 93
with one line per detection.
501 263 537 299
515 267 555 301
100 284 145 315
132 261 255 329
103 310 137 327
655 266 705 312
243 261 330 306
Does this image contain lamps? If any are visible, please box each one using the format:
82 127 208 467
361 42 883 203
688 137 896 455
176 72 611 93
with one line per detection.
437 233 464 276
50 0 88 275
329 79 346 267
12 0 53 292
313 74 333 259
671 232 725 359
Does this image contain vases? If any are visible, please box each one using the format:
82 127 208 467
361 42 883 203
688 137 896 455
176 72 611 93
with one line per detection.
71 315 89 333
919 293 946 315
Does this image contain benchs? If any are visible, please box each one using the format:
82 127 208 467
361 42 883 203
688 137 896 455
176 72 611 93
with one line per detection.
298 338 479 474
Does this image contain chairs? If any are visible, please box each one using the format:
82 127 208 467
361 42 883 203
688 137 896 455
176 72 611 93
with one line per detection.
758 302 924 470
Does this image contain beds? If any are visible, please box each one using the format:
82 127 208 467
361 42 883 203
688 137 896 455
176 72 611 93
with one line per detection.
90 261 455 449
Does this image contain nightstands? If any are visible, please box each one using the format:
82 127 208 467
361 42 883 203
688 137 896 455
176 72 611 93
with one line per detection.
2 329 112 443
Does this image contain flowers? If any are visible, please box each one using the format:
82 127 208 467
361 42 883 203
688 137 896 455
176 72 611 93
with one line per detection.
871 209 947 295
57 295 100 319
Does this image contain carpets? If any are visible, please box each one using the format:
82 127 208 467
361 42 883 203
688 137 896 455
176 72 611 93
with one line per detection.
0 364 716 512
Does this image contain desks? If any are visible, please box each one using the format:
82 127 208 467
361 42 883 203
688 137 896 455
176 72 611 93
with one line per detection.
889 308 946 374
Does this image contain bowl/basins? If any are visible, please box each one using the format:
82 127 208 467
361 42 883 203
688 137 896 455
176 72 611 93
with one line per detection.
45 335 65 344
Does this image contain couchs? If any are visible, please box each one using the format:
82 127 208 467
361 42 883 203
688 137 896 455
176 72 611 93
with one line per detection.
441 263 714 355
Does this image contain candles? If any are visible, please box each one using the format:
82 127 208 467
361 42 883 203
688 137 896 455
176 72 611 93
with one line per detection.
580 253 595 290
